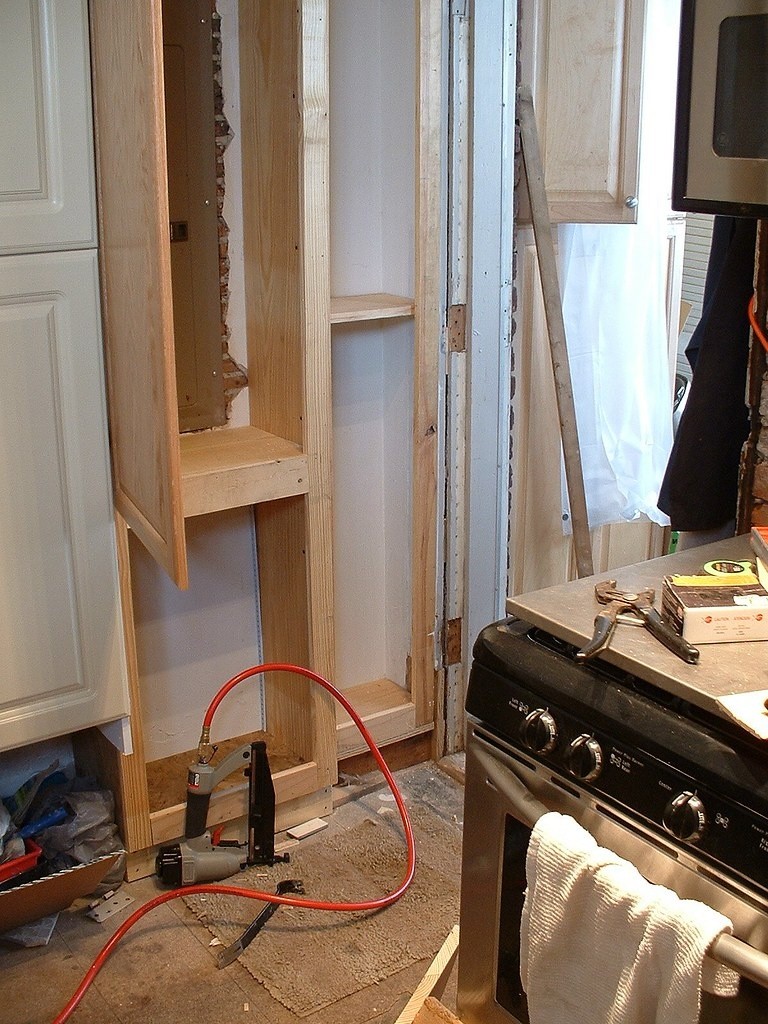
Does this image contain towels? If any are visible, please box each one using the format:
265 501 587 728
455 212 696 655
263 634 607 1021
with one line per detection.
520 810 739 1023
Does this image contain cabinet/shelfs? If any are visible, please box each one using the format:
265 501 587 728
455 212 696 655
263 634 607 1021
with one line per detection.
0 0 128 752
87 0 338 884
330 0 442 781
506 1 687 616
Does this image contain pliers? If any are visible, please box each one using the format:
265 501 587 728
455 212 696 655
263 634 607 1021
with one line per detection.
575 579 700 664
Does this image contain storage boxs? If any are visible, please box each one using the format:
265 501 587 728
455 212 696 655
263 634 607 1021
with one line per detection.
660 571 768 644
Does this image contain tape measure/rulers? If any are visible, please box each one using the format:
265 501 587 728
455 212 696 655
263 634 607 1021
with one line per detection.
699 558 757 576
